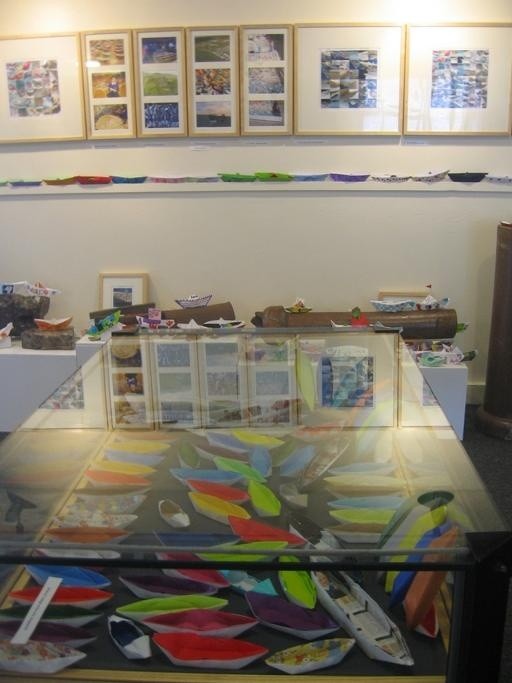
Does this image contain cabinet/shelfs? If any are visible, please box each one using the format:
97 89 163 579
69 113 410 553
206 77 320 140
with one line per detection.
0 323 510 680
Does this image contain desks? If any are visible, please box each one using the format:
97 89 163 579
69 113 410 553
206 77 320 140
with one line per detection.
76 324 118 364
0 333 82 436
403 345 471 441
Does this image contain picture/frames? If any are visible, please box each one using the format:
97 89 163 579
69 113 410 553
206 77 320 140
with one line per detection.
98 269 151 311
99 333 401 433
0 21 512 143
